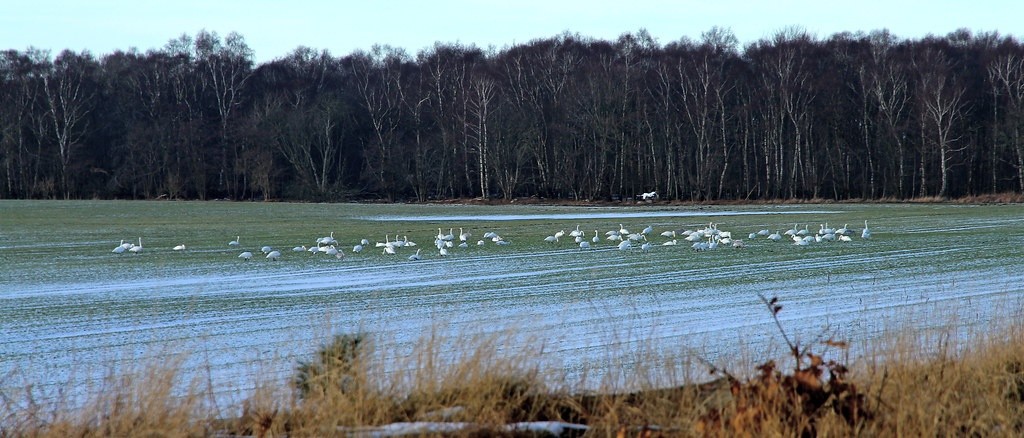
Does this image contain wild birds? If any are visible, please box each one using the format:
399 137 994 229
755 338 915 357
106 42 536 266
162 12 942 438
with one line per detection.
307 231 345 261
360 238 370 246
476 239 484 246
604 223 651 254
660 230 677 246
291 245 306 252
238 250 253 263
748 219 871 246
592 229 600 243
484 231 511 247
680 222 746 252
407 248 422 261
172 244 186 251
112 237 143 254
544 229 565 244
433 228 454 258
568 225 590 250
352 245 362 253
457 227 473 248
228 236 240 246
261 245 281 262
375 234 417 256
